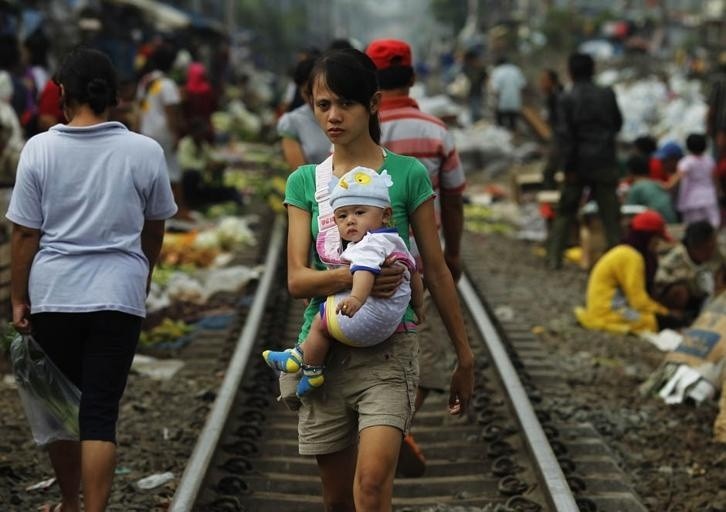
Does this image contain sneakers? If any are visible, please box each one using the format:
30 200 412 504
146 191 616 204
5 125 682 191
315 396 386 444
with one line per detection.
397 436 426 479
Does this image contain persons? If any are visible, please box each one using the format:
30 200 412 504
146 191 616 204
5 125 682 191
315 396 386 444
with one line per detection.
575 210 725 338
262 37 478 512
2 34 225 220
463 47 525 134
6 47 181 511
540 53 725 270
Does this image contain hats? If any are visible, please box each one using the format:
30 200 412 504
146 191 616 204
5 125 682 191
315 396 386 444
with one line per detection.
652 144 683 160
329 166 391 215
630 210 672 243
365 40 411 71
